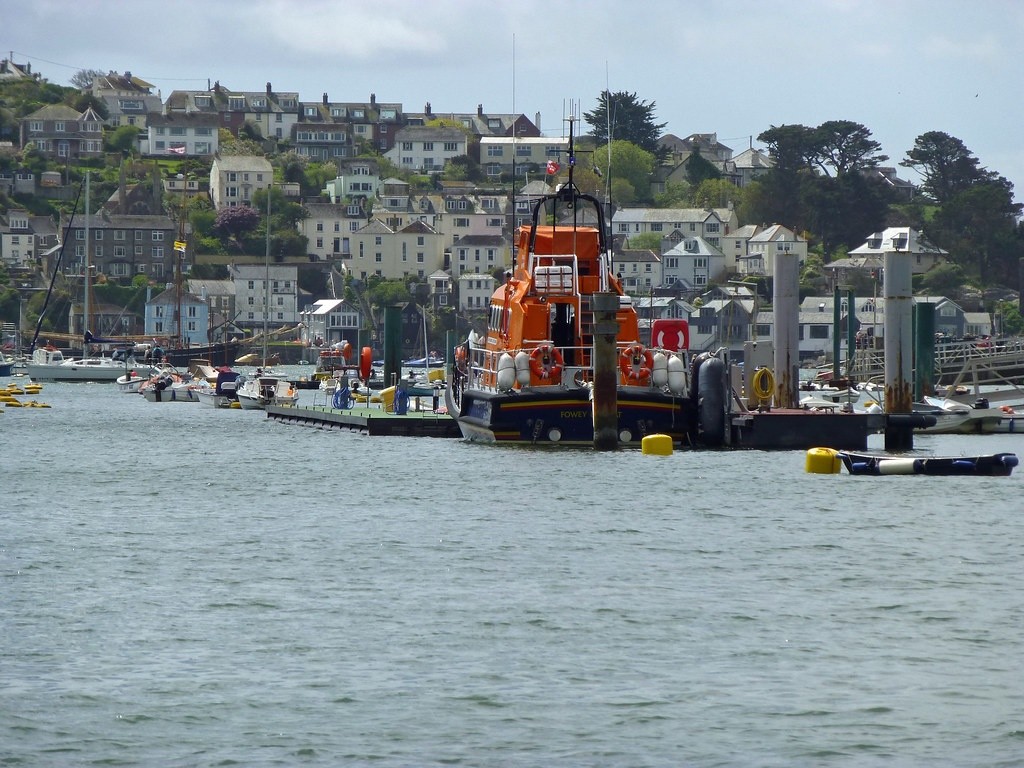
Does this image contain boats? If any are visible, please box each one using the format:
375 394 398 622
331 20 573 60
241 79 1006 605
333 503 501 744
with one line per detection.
444 98 694 444
0 349 447 410
838 450 1019 476
796 371 1024 434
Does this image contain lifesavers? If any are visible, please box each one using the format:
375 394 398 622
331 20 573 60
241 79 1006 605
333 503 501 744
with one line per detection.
619 345 654 380
998 405 1015 415
343 343 352 360
752 367 776 398
455 346 467 371
360 346 372 378
529 346 564 379
